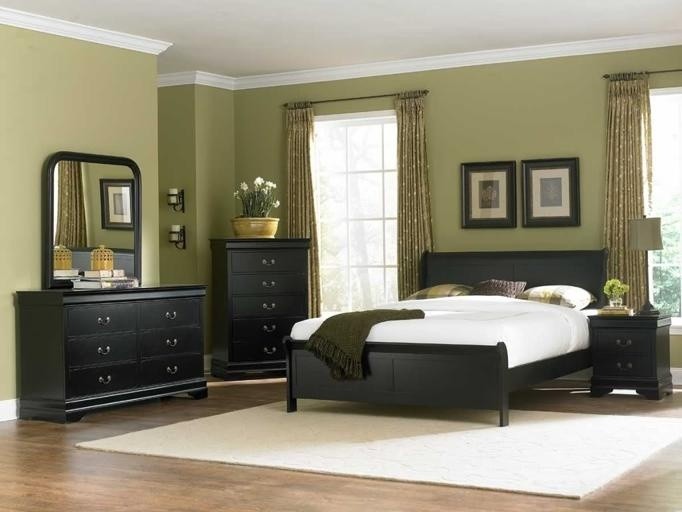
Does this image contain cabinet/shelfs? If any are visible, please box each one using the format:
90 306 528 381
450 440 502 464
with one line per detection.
16 284 207 423
212 239 313 380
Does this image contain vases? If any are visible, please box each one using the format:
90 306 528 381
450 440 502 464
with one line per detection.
230 215 281 238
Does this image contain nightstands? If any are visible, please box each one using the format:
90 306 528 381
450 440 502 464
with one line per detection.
586 314 672 400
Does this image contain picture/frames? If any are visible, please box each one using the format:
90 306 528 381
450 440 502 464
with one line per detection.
456 159 517 228
100 178 138 230
520 156 581 228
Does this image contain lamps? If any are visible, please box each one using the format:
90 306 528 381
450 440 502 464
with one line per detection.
167 187 185 212
168 223 186 249
626 214 663 315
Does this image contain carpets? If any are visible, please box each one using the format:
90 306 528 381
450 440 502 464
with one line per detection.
74 391 682 500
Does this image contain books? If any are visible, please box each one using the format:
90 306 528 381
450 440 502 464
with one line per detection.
599 308 633 316
53 269 84 280
603 305 628 310
71 270 139 291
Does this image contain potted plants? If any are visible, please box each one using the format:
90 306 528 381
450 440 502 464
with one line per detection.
603 278 628 307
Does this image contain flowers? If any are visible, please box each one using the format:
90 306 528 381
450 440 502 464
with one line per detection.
232 175 281 216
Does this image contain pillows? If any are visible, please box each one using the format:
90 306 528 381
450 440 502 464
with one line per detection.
516 285 598 310
401 282 474 301
469 278 527 299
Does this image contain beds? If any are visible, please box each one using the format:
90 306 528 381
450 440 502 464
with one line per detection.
282 249 608 426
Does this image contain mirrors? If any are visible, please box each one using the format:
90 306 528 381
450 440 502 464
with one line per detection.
42 150 143 293
